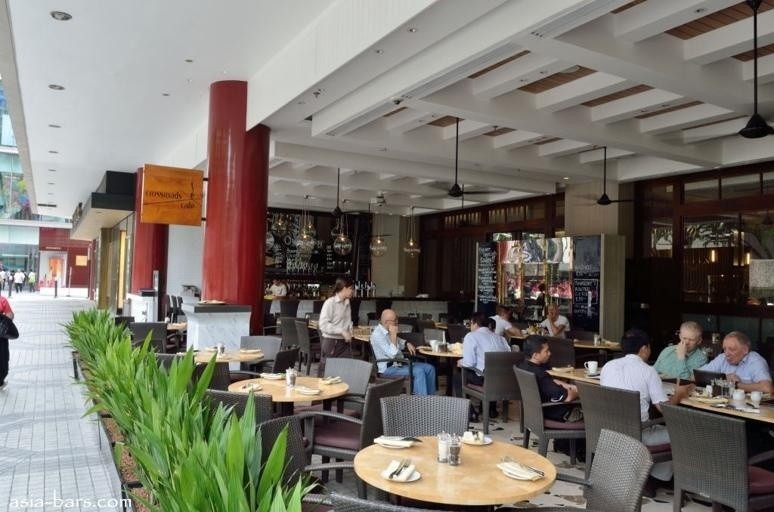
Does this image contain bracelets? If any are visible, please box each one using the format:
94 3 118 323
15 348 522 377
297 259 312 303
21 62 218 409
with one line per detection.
405 341 408 346
735 382 737 389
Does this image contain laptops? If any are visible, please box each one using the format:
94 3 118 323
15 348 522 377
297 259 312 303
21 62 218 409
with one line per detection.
693 368 728 391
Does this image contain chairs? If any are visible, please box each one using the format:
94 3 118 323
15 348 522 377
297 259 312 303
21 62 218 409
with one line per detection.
107 293 774 509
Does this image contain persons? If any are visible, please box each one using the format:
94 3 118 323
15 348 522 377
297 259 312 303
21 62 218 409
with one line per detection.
691 331 774 394
654 320 709 380
489 305 522 336
453 312 511 422
488 317 496 333
319 278 355 373
599 329 675 481
0 281 14 386
371 308 436 396
0 268 36 292
536 302 570 339
266 278 287 315
520 335 585 462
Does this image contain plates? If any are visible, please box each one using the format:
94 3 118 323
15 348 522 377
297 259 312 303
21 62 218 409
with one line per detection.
263 376 282 379
378 435 410 449
197 301 226 305
380 467 421 482
501 467 527 481
240 385 262 391
240 349 260 353
457 435 492 445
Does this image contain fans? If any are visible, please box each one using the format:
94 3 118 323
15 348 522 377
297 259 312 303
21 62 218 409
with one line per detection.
674 0 774 152
422 115 507 201
327 166 361 218
572 144 635 207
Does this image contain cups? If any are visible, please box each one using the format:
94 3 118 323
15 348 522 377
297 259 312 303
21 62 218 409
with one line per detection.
751 390 761 408
733 390 746 410
584 360 598 373
429 340 447 353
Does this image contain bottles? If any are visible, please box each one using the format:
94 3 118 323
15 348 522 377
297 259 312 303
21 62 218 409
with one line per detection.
435 429 452 462
696 379 735 406
521 323 545 335
284 367 295 387
217 342 226 358
448 432 463 464
353 281 375 297
594 334 603 346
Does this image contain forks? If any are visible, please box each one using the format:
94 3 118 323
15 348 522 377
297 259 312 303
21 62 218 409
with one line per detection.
380 435 423 443
388 458 411 480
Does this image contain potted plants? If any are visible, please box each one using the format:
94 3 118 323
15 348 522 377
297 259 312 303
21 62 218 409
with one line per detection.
58 306 153 462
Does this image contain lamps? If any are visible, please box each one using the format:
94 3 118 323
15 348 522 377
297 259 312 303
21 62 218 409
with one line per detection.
401 204 422 259
292 194 316 256
367 201 388 258
332 198 356 256
